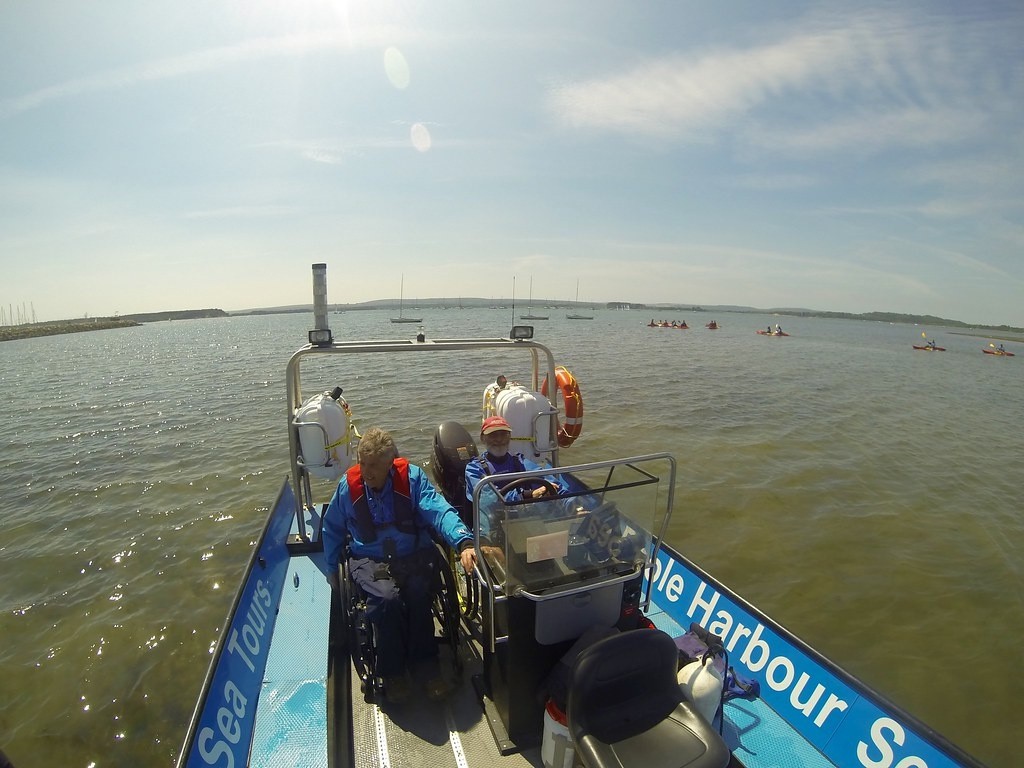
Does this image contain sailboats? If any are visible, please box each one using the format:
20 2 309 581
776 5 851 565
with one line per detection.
390 274 424 322
520 275 550 319
566 277 593 319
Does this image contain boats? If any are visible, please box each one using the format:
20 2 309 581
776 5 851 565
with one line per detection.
705 325 719 329
647 323 689 329
912 345 947 351
983 350 1015 355
755 330 790 336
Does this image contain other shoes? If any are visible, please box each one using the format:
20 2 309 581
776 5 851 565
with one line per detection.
383 675 410 704
414 658 442 684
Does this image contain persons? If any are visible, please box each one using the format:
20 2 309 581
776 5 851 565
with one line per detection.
465 416 579 536
323 429 478 703
928 339 935 347
767 326 771 333
778 327 782 335
710 321 716 327
651 319 687 327
999 344 1004 352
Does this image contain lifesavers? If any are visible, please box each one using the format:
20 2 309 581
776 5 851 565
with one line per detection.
541 364 584 448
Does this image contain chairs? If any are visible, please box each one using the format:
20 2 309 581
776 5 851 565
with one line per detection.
566 629 730 768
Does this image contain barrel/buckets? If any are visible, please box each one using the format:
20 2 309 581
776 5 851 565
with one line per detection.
296 391 352 480
541 698 576 768
482 377 551 464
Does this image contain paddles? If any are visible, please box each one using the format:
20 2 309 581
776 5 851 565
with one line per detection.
921 332 929 343
990 344 1006 354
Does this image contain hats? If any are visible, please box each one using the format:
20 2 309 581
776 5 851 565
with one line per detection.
481 416 512 435
